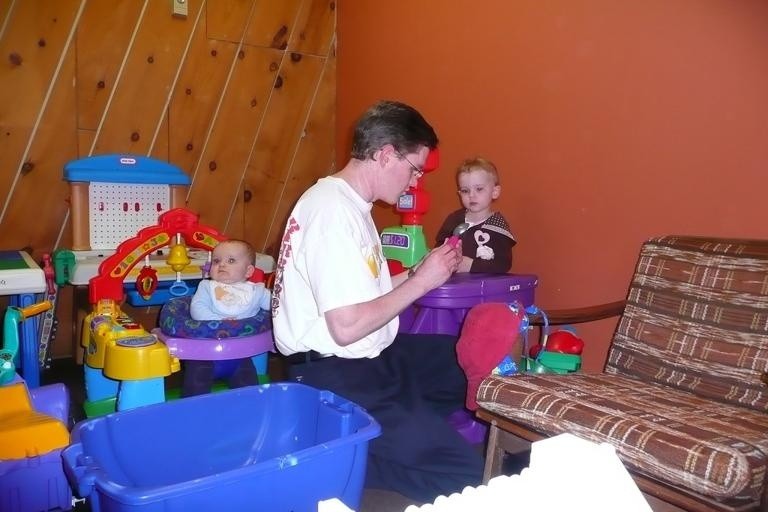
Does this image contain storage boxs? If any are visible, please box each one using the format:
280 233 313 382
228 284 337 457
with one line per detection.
60 379 382 512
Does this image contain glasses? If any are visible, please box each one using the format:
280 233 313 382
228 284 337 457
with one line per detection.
397 150 423 180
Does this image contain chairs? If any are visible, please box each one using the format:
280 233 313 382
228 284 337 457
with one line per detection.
473 234 768 511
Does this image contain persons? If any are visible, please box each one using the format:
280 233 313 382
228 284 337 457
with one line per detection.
433 156 518 275
270 98 486 503
181 238 272 397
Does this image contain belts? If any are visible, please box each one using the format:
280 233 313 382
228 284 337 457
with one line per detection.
282 349 335 368
